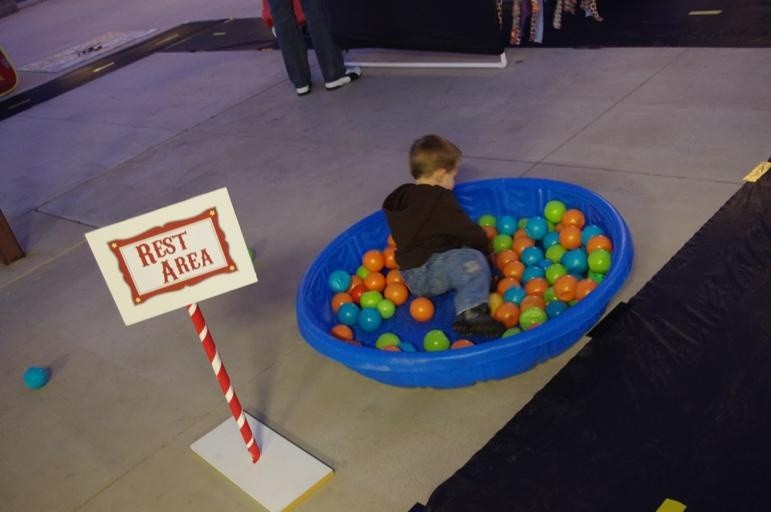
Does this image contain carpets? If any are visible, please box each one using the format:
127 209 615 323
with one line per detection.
413 174 769 509
1 0 771 120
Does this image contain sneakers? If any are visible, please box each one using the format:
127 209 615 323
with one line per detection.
294 79 313 96
322 65 361 91
450 305 506 338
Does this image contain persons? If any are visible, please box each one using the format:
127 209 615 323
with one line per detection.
380 133 504 335
269 1 363 97
